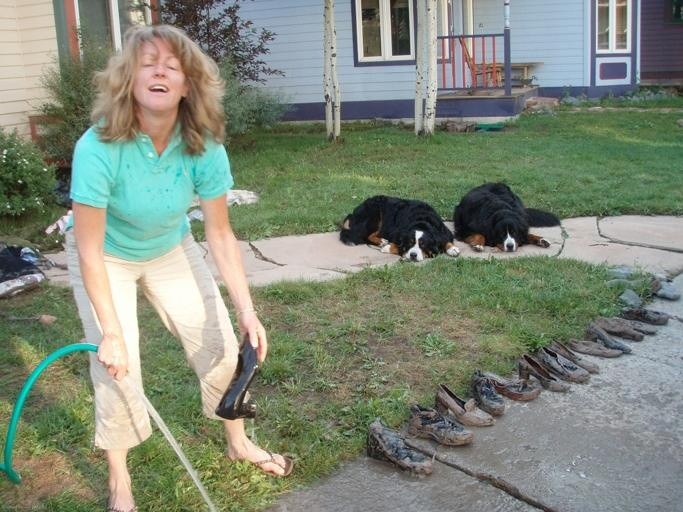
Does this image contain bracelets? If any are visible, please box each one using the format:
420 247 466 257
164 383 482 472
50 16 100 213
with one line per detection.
235 309 257 317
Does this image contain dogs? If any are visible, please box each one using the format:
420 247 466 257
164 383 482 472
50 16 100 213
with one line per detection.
339 195 460 262
453 183 561 252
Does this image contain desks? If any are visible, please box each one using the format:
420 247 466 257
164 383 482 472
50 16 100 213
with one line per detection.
486 63 544 88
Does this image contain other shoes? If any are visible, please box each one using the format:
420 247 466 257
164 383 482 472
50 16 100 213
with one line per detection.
582 323 633 354
597 315 645 342
407 402 475 446
568 338 624 359
612 315 659 335
530 345 591 384
474 369 540 401
620 303 671 325
366 424 430 475
471 374 505 415
549 339 600 376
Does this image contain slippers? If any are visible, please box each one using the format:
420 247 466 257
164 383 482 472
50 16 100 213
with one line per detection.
253 449 294 478
105 496 138 512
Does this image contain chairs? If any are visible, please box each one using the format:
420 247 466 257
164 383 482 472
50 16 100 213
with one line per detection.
458 33 502 88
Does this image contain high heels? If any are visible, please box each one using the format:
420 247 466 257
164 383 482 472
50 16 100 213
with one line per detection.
518 352 571 393
215 332 259 420
436 384 495 427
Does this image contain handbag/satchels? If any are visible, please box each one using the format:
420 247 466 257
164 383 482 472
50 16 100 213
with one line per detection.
0 246 45 299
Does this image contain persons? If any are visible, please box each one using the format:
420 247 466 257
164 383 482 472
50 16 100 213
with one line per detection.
63 23 293 512
